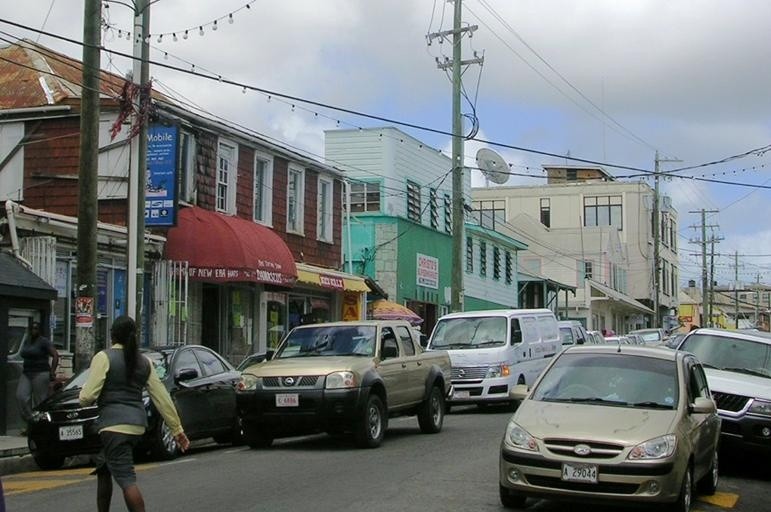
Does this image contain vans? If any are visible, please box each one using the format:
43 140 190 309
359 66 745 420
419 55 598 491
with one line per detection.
425 309 562 406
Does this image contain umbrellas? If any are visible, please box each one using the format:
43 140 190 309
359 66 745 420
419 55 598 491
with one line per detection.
366 298 424 326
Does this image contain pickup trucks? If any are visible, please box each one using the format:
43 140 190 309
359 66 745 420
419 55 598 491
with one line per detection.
235 319 454 447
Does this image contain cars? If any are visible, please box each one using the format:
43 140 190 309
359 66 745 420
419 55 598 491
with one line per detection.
557 319 677 350
26 344 245 467
501 342 725 512
227 346 296 373
680 325 771 450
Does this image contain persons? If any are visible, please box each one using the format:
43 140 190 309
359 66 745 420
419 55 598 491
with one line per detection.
16 321 58 436
79 316 190 512
